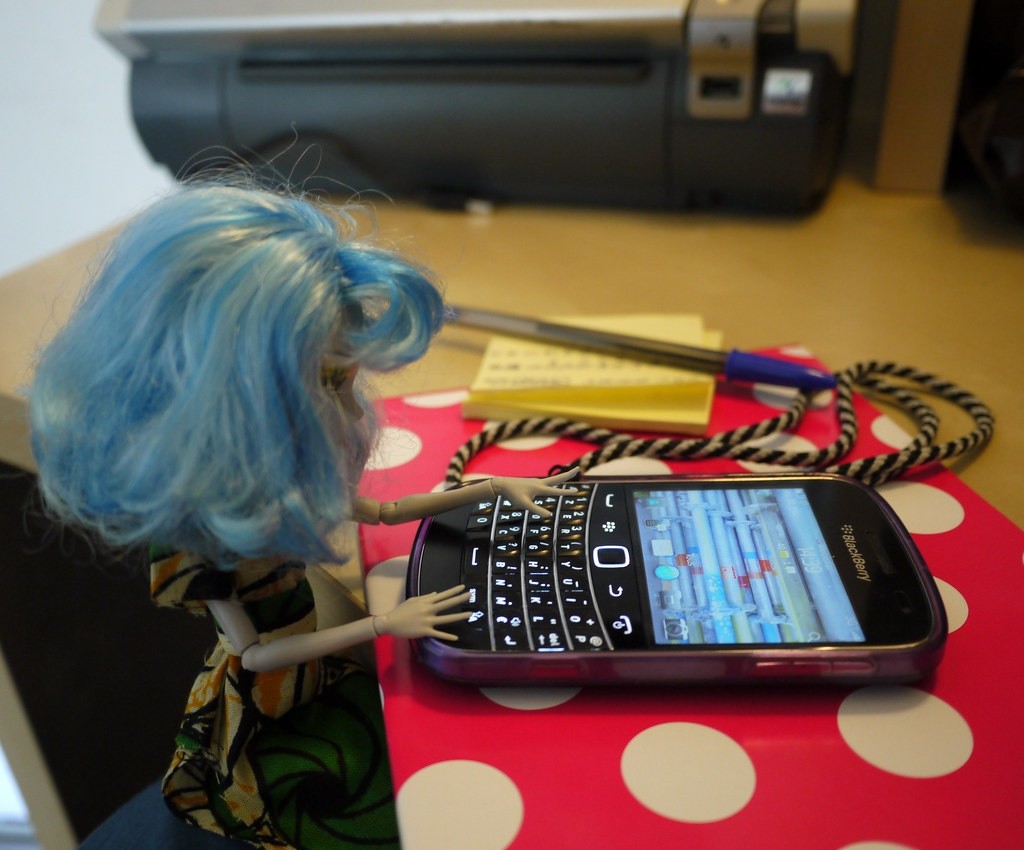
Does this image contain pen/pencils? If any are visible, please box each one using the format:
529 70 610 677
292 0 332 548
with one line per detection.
434 302 838 391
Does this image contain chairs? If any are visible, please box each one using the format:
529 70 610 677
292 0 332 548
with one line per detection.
0 459 254 850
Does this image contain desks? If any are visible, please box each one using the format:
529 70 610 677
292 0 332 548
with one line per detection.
358 346 1024 850
0 177 1024 850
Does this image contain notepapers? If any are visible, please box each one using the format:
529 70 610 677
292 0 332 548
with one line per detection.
465 329 720 437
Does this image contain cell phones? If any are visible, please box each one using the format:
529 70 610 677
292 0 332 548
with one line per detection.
405 473 949 688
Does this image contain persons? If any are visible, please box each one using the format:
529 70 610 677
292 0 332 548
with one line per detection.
25 177 581 850
